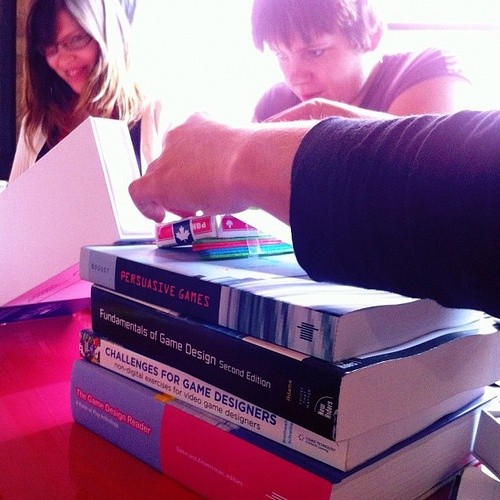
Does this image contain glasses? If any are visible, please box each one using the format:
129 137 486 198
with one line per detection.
39 34 92 57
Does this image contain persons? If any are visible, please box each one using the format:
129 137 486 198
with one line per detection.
249 0 471 123
6 0 173 188
127 97 499 320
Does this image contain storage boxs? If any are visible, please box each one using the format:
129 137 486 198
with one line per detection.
0 116 155 307
155 215 258 247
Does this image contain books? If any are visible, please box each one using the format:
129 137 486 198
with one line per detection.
70 244 500 500
